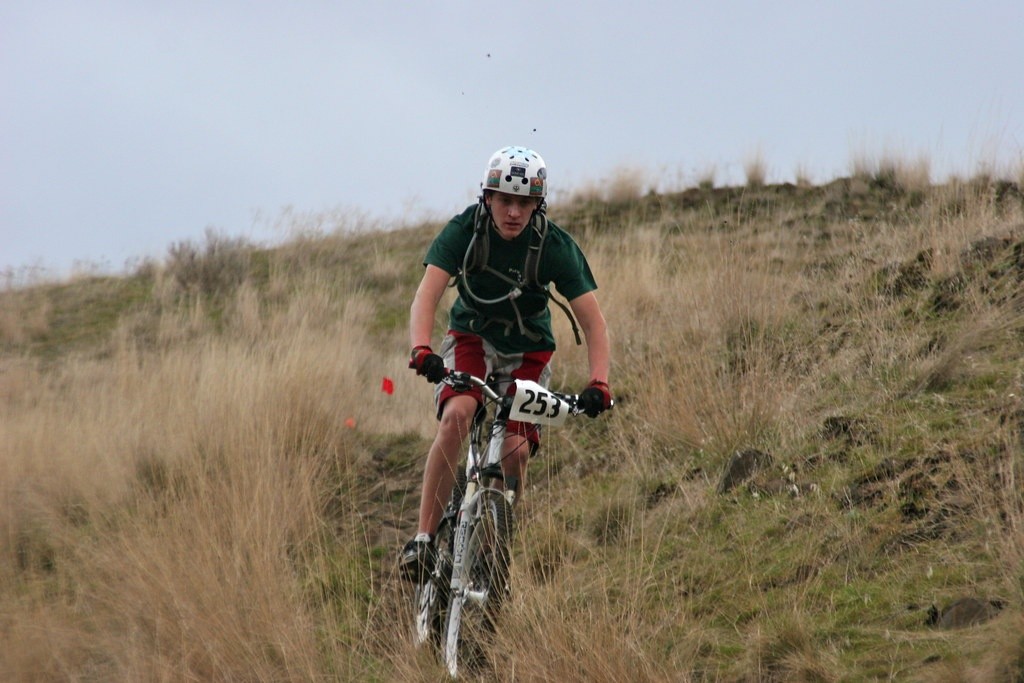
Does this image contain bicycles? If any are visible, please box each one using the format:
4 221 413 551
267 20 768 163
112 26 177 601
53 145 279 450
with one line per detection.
403 359 614 683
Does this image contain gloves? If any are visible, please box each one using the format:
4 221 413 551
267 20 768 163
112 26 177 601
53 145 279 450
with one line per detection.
411 346 444 385
577 379 611 419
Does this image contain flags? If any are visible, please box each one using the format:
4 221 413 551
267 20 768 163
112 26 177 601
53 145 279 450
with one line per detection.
381 377 393 395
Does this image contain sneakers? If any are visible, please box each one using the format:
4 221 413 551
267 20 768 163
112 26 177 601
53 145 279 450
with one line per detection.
472 559 494 597
398 532 439 583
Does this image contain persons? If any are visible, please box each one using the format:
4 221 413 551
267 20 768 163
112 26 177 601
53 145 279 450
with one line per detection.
400 146 611 588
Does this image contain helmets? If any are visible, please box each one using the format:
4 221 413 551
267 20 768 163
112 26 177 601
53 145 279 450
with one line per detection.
481 145 547 198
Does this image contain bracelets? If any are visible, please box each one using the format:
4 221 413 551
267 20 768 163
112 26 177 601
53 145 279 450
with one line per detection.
588 379 608 388
